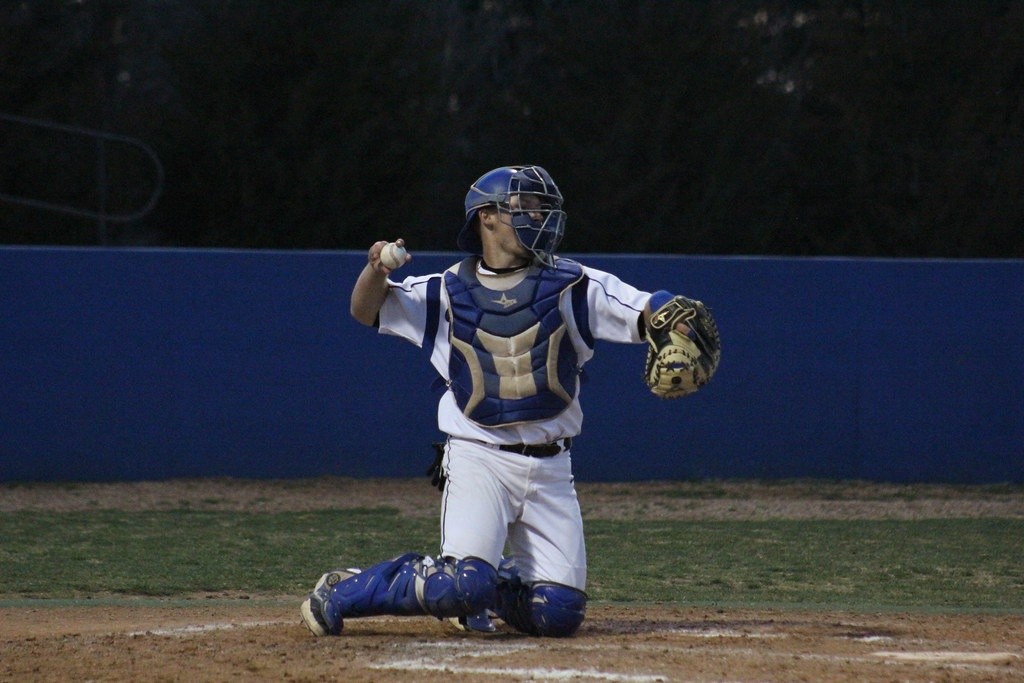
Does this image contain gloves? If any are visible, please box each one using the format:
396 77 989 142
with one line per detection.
425 443 447 491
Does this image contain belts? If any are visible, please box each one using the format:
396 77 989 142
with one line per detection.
477 438 572 457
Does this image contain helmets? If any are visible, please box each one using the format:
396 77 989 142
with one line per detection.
457 167 542 254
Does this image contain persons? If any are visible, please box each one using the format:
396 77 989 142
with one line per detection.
300 166 721 640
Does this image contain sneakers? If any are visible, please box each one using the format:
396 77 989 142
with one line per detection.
300 567 362 637
449 609 506 631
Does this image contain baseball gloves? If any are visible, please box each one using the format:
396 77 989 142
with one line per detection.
639 294 721 401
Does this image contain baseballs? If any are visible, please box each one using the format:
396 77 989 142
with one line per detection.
380 242 408 269
